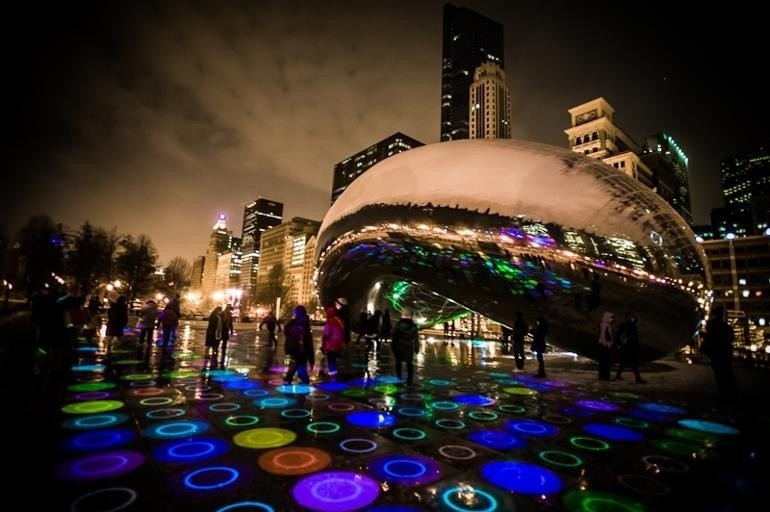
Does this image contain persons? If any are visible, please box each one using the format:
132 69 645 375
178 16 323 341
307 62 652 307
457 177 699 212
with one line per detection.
444 319 454 336
259 312 282 348
689 303 737 389
284 305 314 386
88 293 180 345
511 312 547 378
206 303 233 355
320 297 420 386
598 311 648 385
